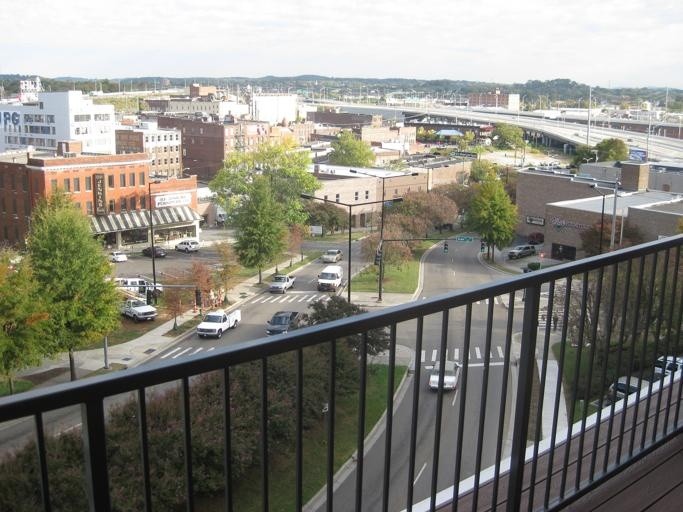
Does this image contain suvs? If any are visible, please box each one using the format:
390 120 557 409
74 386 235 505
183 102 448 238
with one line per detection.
175 240 201 252
507 244 536 258
605 381 639 406
652 354 683 376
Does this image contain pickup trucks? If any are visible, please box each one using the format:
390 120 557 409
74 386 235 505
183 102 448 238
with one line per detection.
195 306 242 337
268 272 297 294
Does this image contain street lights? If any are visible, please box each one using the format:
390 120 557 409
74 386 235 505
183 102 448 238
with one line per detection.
295 192 410 304
255 166 281 180
584 180 607 254
147 179 165 305
348 167 422 303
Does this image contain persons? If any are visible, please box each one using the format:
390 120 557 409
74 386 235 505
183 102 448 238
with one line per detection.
552 313 558 331
207 288 215 309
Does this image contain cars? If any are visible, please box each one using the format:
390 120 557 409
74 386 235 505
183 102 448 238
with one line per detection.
427 357 462 392
141 245 168 258
265 310 301 337
323 248 344 264
106 250 128 263
526 231 545 244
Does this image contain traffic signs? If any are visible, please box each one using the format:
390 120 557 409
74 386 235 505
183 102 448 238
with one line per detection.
456 235 473 242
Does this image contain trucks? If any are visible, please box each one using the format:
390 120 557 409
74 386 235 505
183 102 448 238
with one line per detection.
317 265 345 291
215 206 230 228
105 276 163 322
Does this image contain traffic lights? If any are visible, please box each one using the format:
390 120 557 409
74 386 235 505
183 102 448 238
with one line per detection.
444 242 448 254
480 238 486 252
